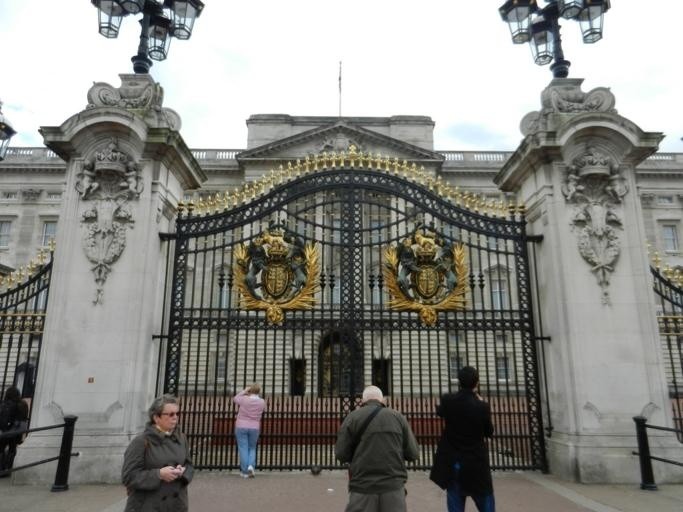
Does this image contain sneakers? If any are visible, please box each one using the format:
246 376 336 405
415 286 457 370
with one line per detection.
239 472 249 478
247 464 255 478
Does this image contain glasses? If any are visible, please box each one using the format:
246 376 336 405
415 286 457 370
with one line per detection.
159 411 181 417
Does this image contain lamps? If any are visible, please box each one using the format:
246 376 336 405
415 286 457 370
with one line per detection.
89 0 206 74
495 0 611 76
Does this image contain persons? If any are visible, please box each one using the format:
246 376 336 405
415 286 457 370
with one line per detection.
428 365 495 511
233 382 265 477
334 385 420 512
121 395 194 512
0 386 28 478
